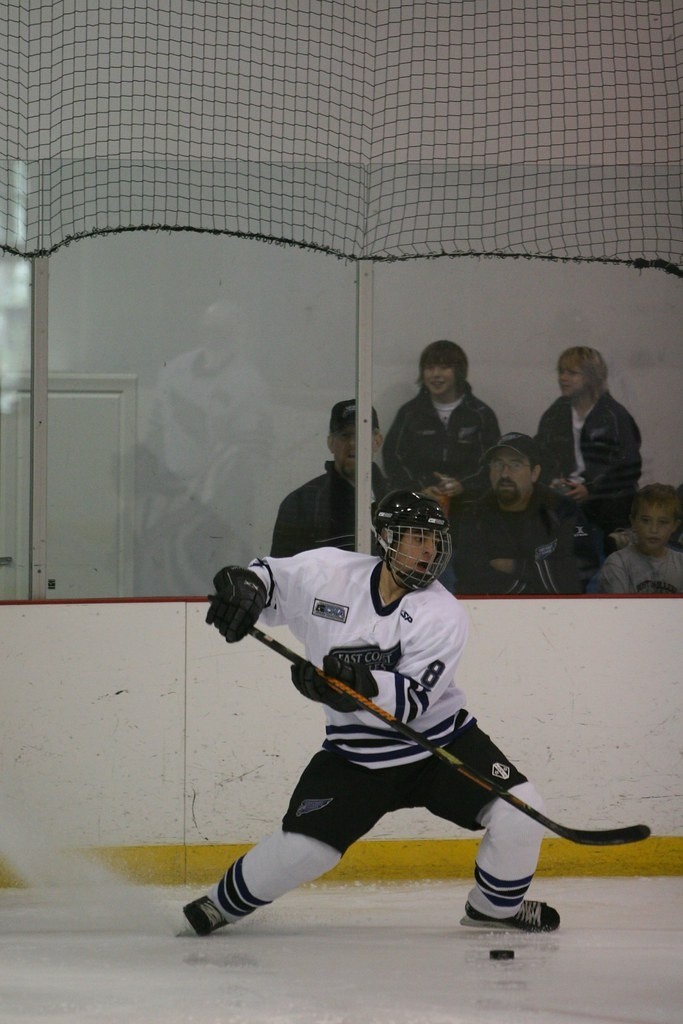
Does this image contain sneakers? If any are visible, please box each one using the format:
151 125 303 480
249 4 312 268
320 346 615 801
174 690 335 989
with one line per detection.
182 895 228 936
460 885 561 933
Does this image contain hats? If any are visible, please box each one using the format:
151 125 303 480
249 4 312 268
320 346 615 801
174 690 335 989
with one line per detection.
481 431 540 460
329 399 380 432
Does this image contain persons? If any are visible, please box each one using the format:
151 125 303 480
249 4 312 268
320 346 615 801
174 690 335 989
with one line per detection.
532 346 643 583
457 431 605 594
182 488 561 937
587 481 683 594
381 340 502 594
268 398 395 557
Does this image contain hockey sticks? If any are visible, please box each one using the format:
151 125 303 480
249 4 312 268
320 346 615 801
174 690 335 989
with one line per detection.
206 590 653 848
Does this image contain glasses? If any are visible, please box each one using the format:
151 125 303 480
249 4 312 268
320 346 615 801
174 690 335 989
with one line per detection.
490 461 531 471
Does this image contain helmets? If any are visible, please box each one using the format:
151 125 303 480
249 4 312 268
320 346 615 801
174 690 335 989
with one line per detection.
375 490 454 591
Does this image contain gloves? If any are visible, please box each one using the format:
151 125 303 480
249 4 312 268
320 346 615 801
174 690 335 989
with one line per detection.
290 655 379 712
205 566 268 642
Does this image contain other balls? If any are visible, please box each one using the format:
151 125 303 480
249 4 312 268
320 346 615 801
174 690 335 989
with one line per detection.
488 949 515 961
